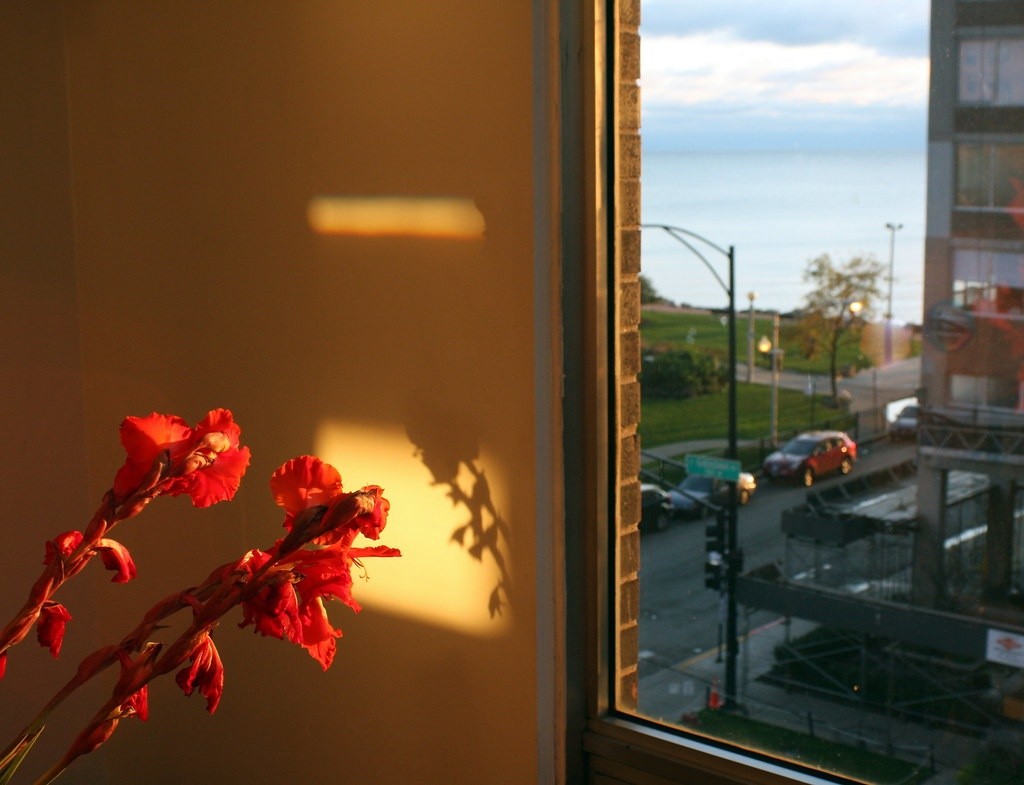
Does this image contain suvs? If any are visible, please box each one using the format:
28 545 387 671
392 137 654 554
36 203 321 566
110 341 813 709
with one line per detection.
764 429 858 488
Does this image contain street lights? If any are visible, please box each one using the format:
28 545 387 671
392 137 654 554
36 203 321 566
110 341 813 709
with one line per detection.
758 313 779 450
746 291 759 384
884 222 904 364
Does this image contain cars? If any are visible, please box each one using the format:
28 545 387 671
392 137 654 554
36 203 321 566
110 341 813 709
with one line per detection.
667 469 756 519
639 484 673 534
886 396 920 439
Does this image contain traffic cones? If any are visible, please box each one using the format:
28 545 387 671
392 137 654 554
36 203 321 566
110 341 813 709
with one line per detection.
708 678 722 711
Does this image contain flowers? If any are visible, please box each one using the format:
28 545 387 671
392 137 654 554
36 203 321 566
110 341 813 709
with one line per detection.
0 408 403 785
